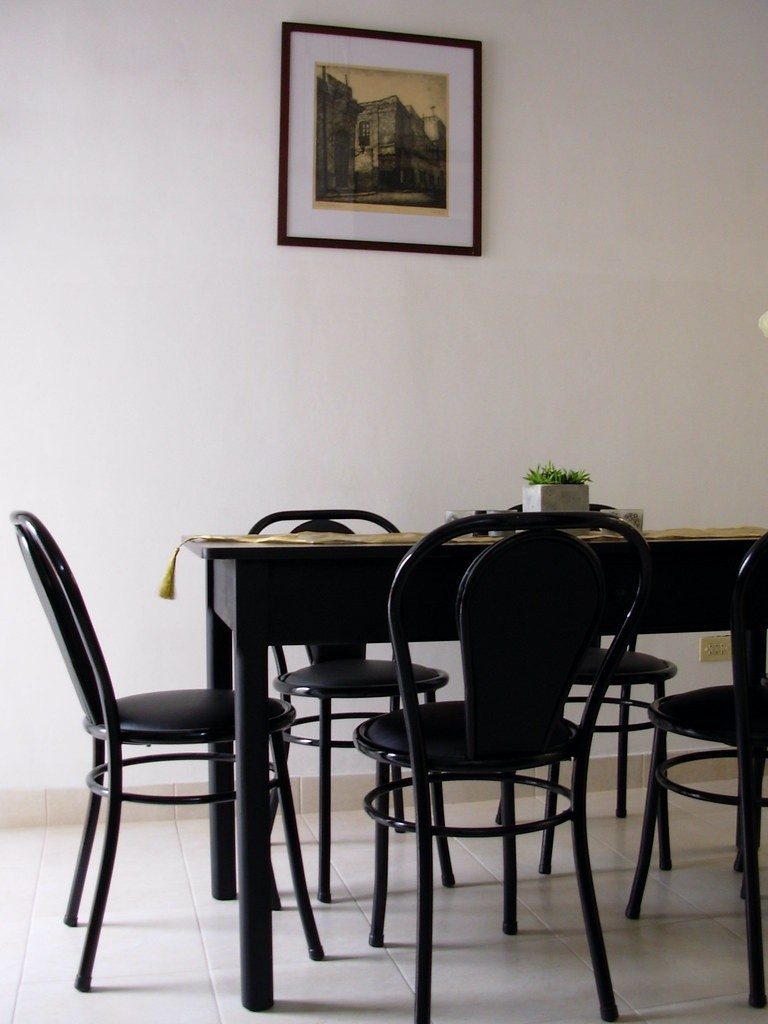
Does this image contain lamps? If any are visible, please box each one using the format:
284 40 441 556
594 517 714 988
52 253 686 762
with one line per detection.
356 514 658 1024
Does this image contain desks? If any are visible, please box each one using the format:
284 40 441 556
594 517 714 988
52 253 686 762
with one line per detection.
183 526 768 1014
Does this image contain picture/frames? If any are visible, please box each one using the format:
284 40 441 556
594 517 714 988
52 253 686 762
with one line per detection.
277 20 487 258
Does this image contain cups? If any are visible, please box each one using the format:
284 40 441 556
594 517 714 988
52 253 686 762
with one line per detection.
487 509 517 538
598 508 644 537
445 510 475 538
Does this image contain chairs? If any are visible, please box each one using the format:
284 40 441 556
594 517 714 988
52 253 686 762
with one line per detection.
625 532 768 1006
475 504 678 870
11 511 324 995
251 508 456 886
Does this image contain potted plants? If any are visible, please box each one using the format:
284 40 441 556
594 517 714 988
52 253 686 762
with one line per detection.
521 462 594 536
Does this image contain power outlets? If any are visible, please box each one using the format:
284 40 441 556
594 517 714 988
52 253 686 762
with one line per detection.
699 636 731 662
